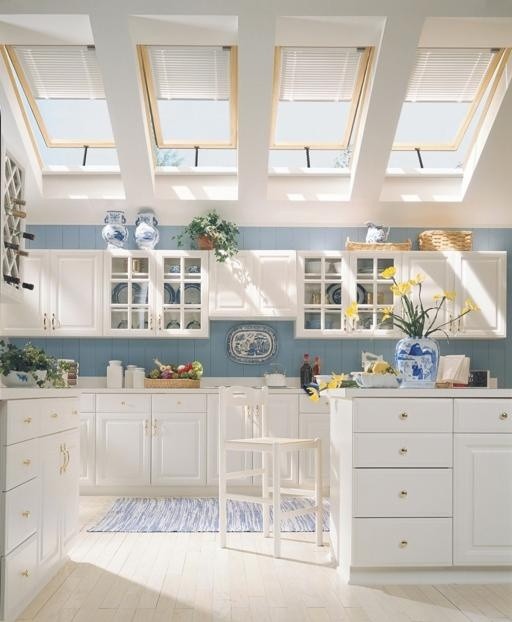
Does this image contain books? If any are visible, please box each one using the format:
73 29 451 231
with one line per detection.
436 354 471 386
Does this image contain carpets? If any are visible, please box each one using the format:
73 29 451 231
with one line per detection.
88 497 327 534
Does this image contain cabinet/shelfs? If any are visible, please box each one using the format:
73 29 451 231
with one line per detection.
2 149 27 303
82 388 328 498
330 399 512 586
0 386 82 620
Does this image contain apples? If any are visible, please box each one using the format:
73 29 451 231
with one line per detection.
150 361 197 379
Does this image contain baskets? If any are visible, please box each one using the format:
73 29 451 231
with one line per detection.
144 378 200 389
418 230 473 252
346 236 413 251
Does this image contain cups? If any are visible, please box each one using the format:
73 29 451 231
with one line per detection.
333 262 342 273
169 264 200 274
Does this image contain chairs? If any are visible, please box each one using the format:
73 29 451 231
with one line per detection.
216 388 324 557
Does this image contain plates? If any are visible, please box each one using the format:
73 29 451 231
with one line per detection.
224 324 280 366
164 283 176 304
356 266 389 279
111 282 142 304
117 319 201 329
183 285 201 305
326 282 368 304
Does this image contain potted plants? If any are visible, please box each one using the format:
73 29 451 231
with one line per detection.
0 338 79 387
169 208 241 263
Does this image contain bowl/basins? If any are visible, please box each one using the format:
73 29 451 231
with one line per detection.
308 261 330 273
353 372 404 389
314 374 332 385
308 319 332 329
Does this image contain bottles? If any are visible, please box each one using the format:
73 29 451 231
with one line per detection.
106 360 124 389
300 352 320 390
124 364 146 389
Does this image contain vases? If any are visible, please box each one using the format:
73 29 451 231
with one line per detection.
396 337 440 387
106 359 145 389
102 210 161 248
